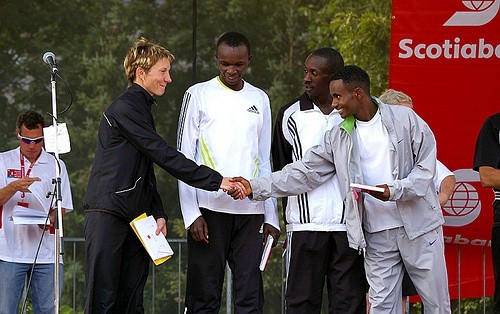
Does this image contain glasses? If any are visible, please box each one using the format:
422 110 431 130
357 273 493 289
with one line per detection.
17 132 44 144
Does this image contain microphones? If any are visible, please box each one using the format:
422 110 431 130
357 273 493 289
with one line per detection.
42 51 58 73
